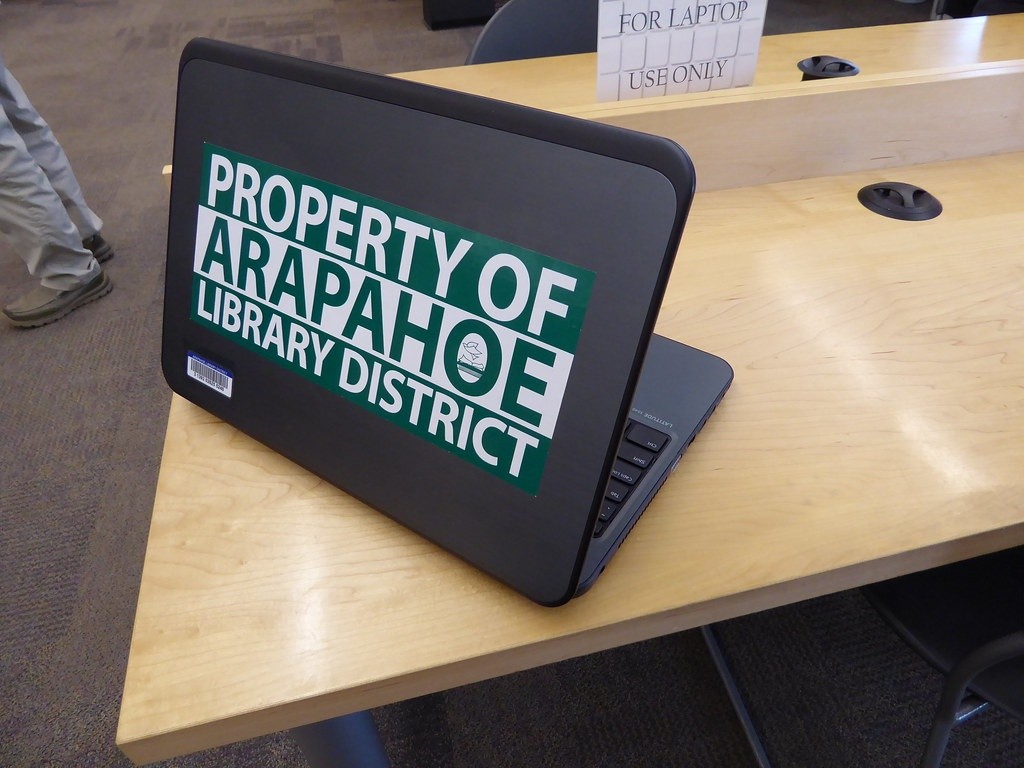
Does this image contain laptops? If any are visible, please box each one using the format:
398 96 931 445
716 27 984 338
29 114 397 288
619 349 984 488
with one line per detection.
160 38 733 608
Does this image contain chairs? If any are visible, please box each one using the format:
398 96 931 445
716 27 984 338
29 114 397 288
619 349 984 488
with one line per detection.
461 0 599 66
698 543 1024 768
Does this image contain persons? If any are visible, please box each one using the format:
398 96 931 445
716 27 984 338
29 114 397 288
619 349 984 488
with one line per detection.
0 65 113 328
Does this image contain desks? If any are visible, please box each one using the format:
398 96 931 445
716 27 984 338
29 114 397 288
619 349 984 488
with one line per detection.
113 10 1024 768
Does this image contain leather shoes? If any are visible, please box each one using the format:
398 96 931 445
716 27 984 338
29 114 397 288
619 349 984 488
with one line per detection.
82 232 114 263
1 269 113 328
